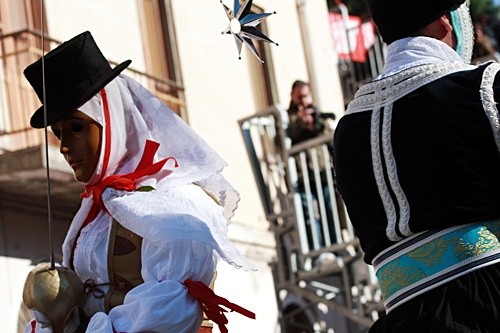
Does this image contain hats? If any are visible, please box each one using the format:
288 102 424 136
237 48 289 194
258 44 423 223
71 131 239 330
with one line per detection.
23 31 132 128
368 0 468 45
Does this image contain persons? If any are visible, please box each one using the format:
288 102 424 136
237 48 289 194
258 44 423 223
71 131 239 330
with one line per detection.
286 80 351 269
333 0 500 333
22 30 217 333
